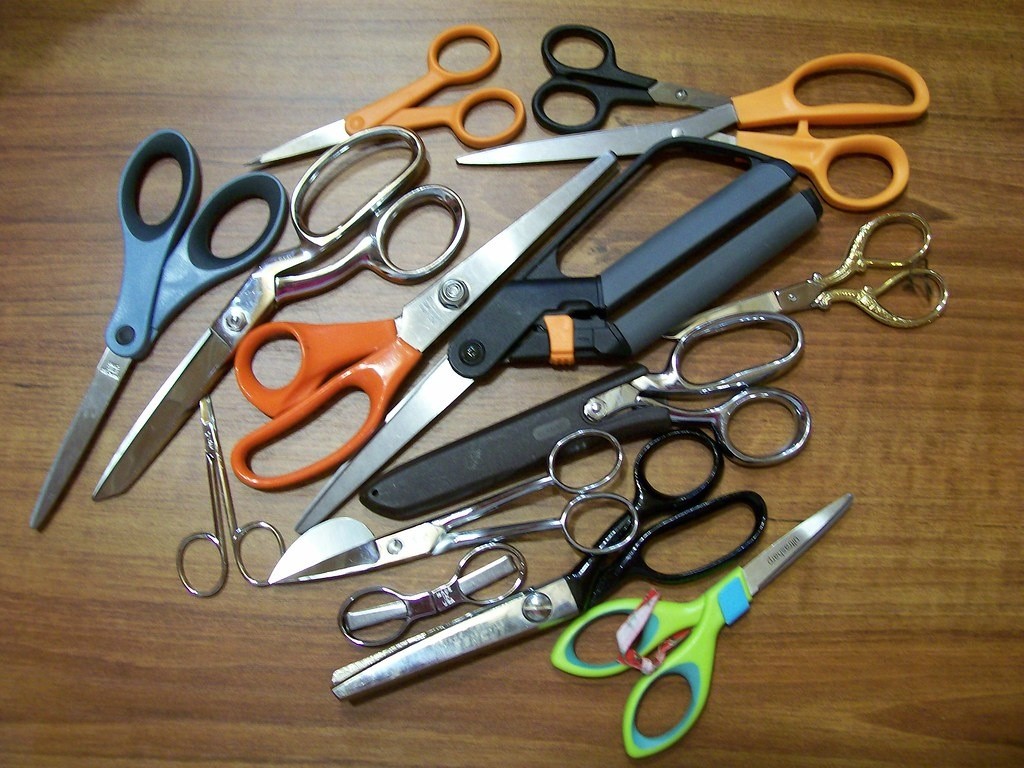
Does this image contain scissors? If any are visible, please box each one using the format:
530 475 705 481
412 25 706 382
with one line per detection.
26 10 953 759
